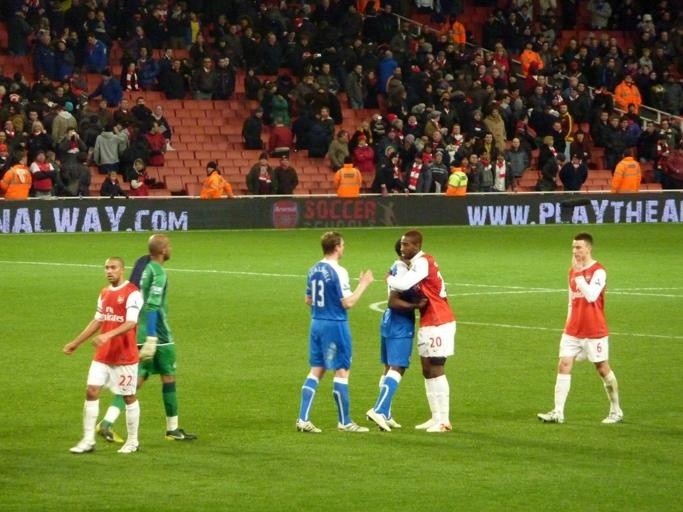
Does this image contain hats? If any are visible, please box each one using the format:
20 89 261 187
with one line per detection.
643 14 652 22
361 121 369 127
344 157 352 163
431 111 442 117
207 161 216 169
256 107 264 113
557 153 565 160
260 153 267 160
450 160 461 167
387 114 397 122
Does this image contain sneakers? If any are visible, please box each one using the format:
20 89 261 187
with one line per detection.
384 416 401 428
95 420 124 443
165 429 197 441
337 422 369 432
536 411 564 424
296 418 322 433
117 442 139 453
426 422 453 432
416 418 437 429
69 439 96 453
366 408 392 432
601 410 623 424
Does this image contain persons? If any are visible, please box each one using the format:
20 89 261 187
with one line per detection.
537 233 623 423
1 0 683 202
64 257 144 454
366 239 429 432
385 230 456 433
296 232 374 433
96 234 198 440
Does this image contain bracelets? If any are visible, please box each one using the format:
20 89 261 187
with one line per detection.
574 272 583 277
